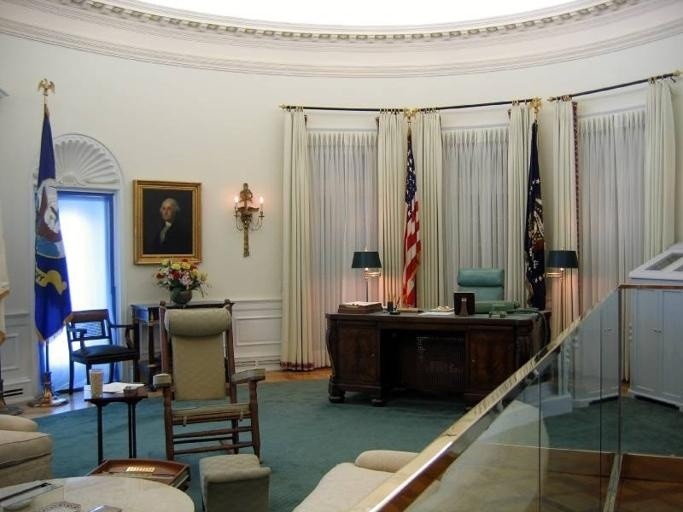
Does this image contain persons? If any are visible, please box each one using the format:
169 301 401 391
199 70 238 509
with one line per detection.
151 197 181 242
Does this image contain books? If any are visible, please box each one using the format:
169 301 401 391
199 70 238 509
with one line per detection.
339 300 383 315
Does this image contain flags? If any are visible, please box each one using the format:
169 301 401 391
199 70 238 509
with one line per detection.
35 115 71 347
403 140 422 308
523 126 547 311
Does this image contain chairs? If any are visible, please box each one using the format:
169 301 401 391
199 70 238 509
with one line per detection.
154 301 265 461
457 269 504 301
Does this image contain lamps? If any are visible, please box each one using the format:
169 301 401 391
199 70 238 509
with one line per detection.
545 250 577 332
351 248 382 302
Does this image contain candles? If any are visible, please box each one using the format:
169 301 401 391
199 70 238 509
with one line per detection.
235 196 239 214
260 197 264 215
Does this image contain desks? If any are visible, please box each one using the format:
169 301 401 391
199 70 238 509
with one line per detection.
324 301 551 409
84 383 148 463
0 476 196 512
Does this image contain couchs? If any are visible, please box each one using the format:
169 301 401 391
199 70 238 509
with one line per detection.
0 414 54 487
198 454 270 511
293 450 417 512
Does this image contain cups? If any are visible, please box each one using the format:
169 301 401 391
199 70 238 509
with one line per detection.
89 371 103 398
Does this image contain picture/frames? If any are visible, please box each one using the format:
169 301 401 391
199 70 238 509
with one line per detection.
133 180 203 266
